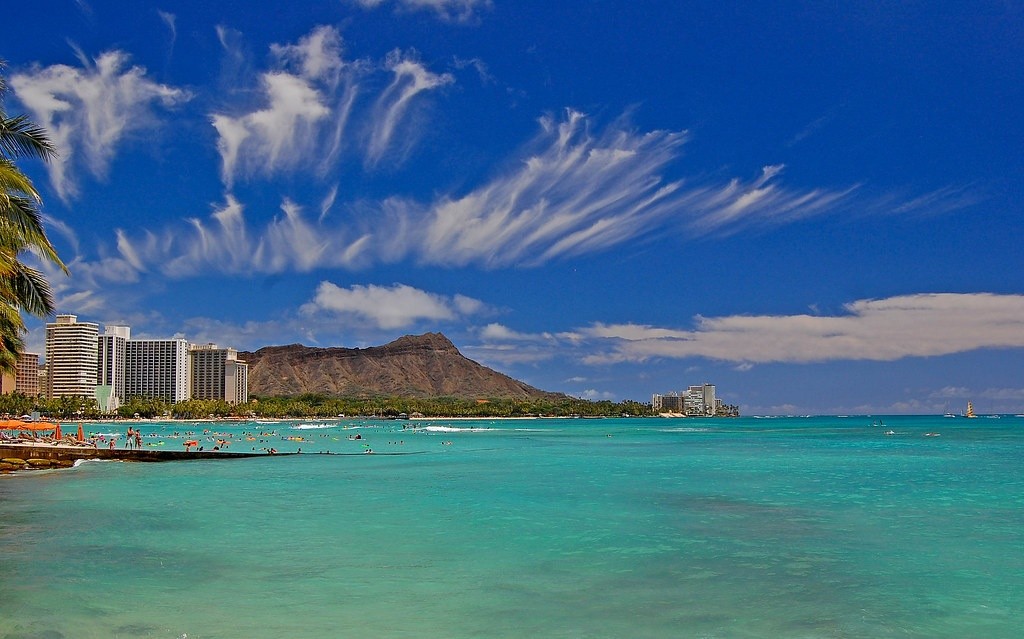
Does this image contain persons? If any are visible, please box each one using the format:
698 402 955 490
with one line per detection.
0 410 500 463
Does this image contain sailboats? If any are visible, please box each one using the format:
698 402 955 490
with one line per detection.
966 400 978 418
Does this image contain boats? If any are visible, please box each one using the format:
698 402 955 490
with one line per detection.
883 430 895 435
943 412 956 418
926 432 941 436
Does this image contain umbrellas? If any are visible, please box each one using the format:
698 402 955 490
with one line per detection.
33 422 56 437
55 422 62 440
0 420 25 436
25 421 39 436
18 423 31 434
77 423 84 441
